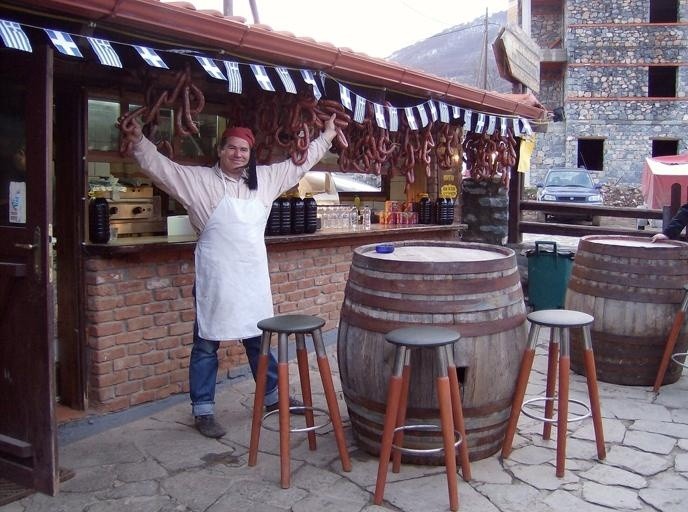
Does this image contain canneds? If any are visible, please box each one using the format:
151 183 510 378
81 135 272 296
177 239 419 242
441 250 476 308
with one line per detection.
379 200 418 224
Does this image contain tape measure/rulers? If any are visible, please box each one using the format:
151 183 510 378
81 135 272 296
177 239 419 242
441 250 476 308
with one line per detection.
376 246 395 253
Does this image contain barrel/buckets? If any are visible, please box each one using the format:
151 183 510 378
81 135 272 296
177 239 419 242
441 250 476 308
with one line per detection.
337 239 527 466
417 191 432 224
435 194 455 226
564 232 688 386
280 191 295 233
289 191 307 233
270 198 281 234
265 215 271 234
303 191 316 233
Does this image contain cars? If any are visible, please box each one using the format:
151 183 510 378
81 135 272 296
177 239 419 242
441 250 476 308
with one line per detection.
536 166 603 227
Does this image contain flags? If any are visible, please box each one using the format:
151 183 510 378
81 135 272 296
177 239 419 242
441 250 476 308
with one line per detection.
223 60 242 94
299 69 327 101
132 45 170 69
44 27 83 57
275 67 298 94
87 37 123 69
1 19 33 53
249 63 276 91
195 55 228 80
339 85 533 140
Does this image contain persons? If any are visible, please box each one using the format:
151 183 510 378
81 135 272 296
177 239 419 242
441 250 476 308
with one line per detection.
116 107 339 439
650 201 688 244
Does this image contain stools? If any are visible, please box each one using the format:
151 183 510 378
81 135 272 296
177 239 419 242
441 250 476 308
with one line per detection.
500 307 606 478
374 326 472 511
653 283 688 395
247 313 354 489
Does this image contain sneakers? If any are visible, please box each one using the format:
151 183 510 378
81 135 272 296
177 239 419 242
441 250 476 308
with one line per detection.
265 395 306 416
195 414 226 439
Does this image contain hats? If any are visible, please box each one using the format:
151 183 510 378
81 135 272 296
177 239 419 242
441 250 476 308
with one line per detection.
221 125 256 150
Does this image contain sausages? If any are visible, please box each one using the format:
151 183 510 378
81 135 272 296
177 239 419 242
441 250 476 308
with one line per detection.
122 65 517 193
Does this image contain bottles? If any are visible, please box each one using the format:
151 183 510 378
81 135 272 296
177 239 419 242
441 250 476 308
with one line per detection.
417 192 455 226
316 203 371 230
264 191 318 236
